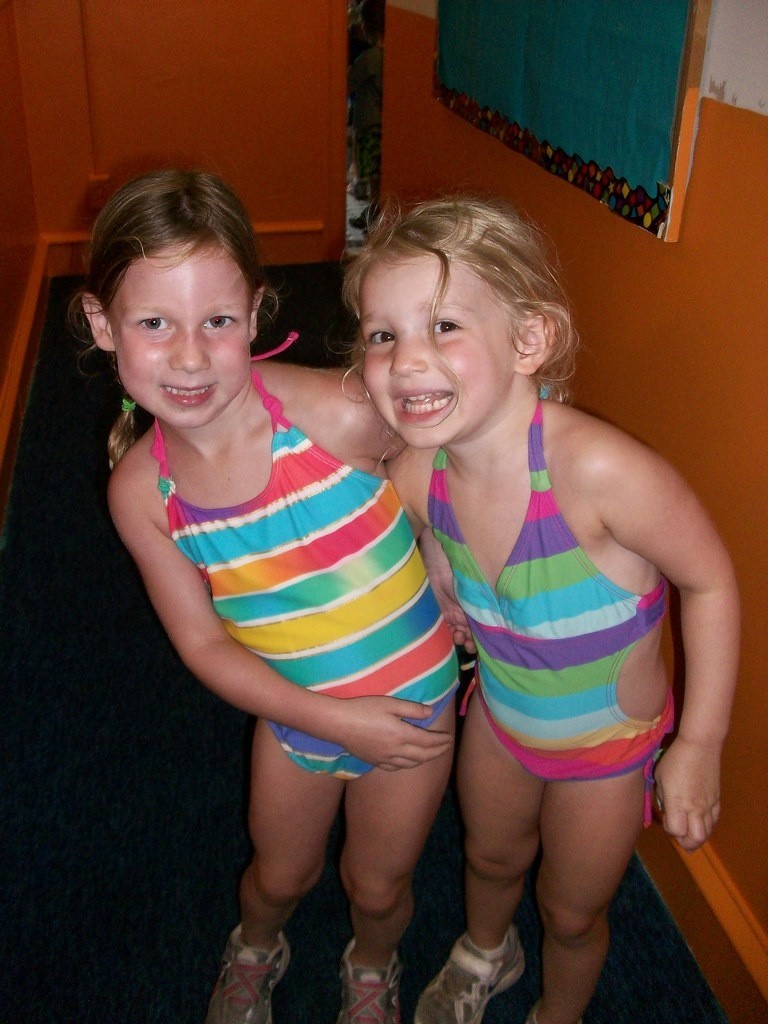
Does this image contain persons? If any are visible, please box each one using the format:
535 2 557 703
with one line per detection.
68 171 459 1024
344 201 741 1024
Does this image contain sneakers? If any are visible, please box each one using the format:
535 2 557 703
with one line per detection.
206 922 290 1024
414 923 524 1024
337 937 401 1024
526 998 582 1024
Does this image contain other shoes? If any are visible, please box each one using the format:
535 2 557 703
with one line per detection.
346 178 370 199
348 202 381 228
